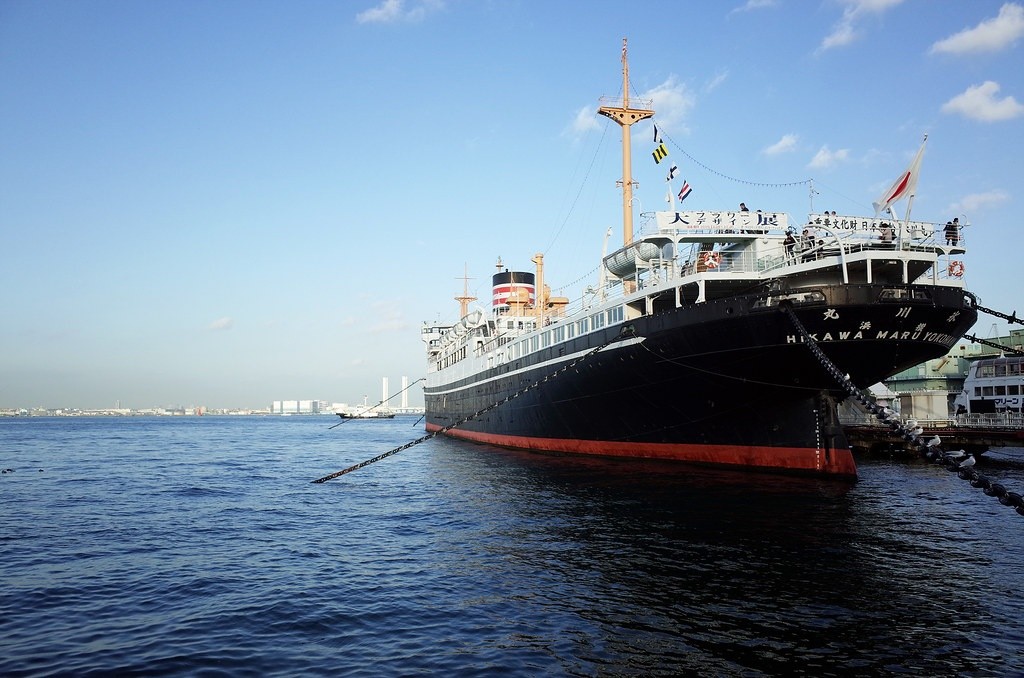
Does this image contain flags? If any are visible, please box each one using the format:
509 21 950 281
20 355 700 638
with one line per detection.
653 124 663 143
678 179 692 203
869 134 928 226
652 143 668 165
666 161 680 183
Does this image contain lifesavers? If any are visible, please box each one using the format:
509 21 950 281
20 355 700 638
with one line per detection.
950 260 965 277
704 251 721 268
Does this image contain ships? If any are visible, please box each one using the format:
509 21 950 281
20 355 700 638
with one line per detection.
421 36 979 497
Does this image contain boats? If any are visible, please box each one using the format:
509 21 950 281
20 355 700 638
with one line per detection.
335 398 396 419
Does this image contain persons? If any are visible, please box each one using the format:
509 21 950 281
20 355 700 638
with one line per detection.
875 223 892 244
783 232 796 266
681 260 692 277
799 230 812 263
956 404 968 424
653 270 660 286
755 210 769 234
816 240 824 259
824 211 830 214
831 211 836 214
740 203 749 234
1007 406 1013 425
943 218 963 246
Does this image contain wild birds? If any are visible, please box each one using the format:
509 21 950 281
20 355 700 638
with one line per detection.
883 404 976 468
